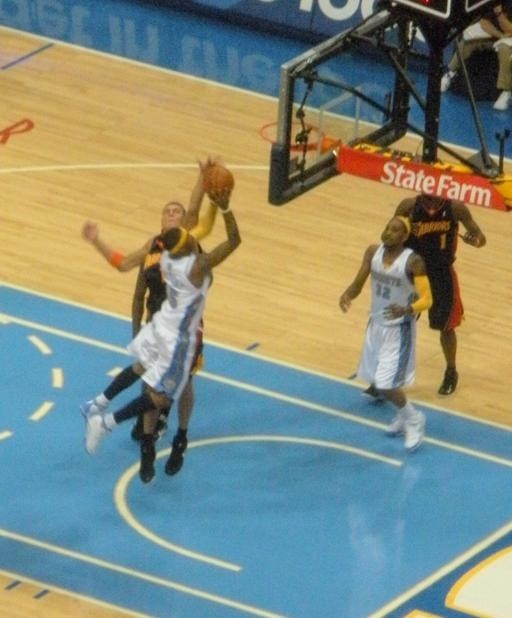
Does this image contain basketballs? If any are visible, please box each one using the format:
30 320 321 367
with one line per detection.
199 166 232 195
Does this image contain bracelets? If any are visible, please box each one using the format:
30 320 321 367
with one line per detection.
475 238 480 247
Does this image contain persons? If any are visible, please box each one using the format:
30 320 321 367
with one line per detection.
336 215 434 453
358 189 488 403
130 200 219 485
440 0 511 111
218 207 232 215
81 153 223 440
77 184 242 461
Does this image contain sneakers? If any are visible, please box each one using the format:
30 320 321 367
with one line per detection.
492 91 511 111
441 70 457 92
81 396 187 480
363 375 457 451
